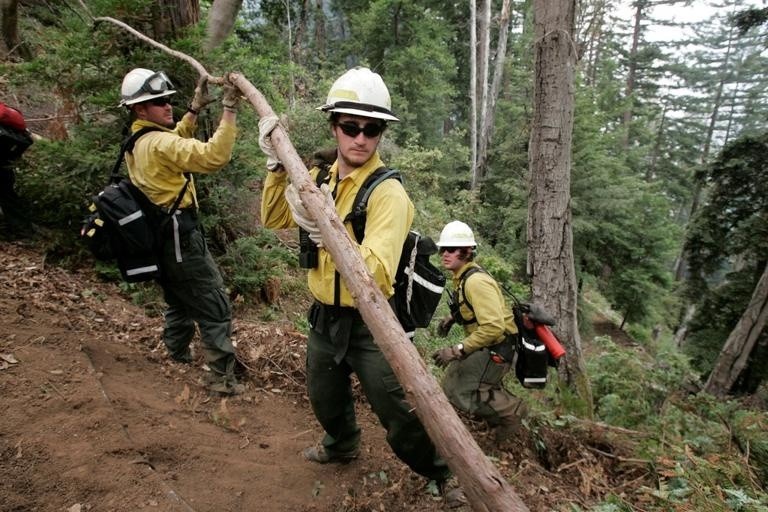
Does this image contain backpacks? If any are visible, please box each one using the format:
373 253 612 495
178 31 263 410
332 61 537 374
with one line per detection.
315 162 446 328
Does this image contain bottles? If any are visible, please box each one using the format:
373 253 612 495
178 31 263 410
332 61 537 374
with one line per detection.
533 321 565 360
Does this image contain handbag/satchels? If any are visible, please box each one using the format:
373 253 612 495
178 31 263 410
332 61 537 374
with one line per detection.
79 126 190 284
447 266 566 389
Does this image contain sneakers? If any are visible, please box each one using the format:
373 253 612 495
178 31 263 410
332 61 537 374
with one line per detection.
210 356 250 376
169 353 204 363
441 475 465 508
495 405 529 441
304 445 361 464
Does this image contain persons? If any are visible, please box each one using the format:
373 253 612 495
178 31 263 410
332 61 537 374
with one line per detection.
432 219 535 449
117 67 248 382
257 66 468 510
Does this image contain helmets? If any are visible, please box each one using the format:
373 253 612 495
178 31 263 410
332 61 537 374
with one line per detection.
315 68 400 121
117 68 177 108
435 221 477 247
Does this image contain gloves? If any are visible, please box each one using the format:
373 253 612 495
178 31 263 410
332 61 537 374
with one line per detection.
284 183 335 248
191 75 218 111
258 116 280 170
222 71 243 108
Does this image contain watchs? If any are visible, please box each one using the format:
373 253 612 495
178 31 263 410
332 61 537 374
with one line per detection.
456 342 469 360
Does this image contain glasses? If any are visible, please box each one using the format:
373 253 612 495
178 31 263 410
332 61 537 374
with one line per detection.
122 71 175 100
441 247 463 253
335 120 386 137
